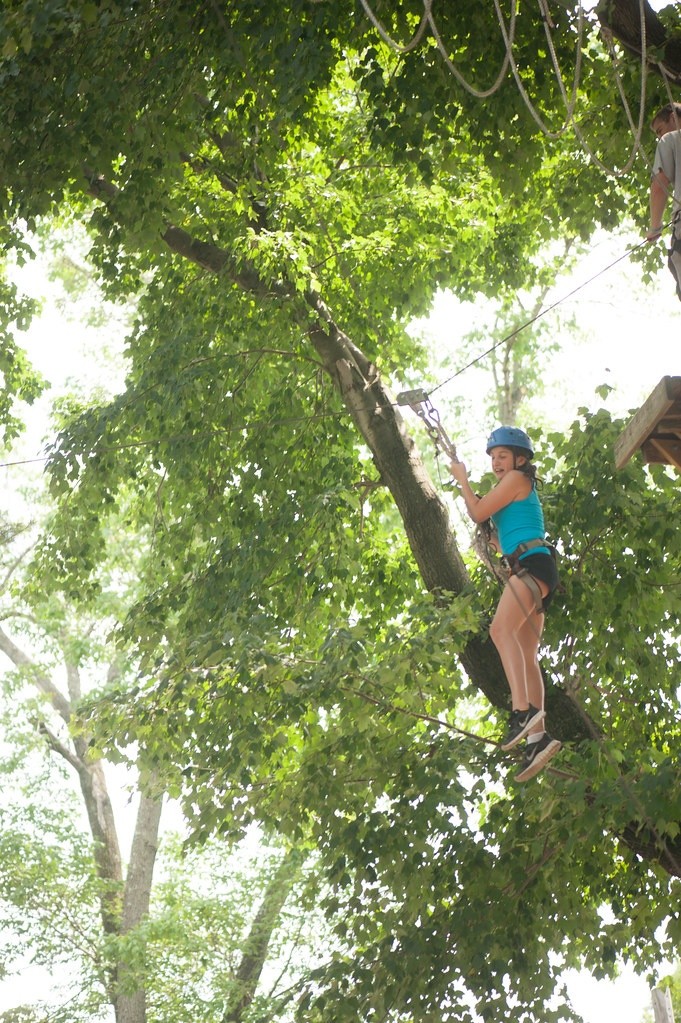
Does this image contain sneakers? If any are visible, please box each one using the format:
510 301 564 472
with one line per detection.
500 703 547 751
513 733 562 783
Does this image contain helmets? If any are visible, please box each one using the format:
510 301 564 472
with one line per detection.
487 425 535 458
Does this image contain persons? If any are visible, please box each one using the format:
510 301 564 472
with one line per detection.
450 426 562 782
645 101 681 302
